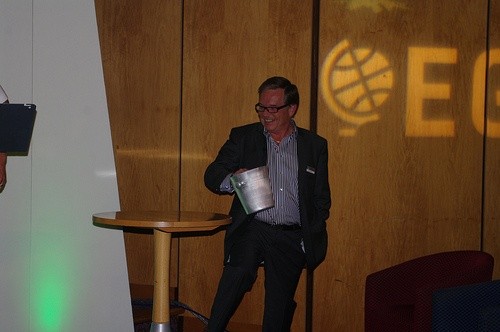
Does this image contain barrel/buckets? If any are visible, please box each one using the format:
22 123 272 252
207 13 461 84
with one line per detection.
229 166 274 215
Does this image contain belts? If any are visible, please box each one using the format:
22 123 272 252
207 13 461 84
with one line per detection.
263 221 301 230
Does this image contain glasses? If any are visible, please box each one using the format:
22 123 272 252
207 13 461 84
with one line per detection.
254 102 291 113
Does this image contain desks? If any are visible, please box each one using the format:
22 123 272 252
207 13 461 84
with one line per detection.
93 210 232 332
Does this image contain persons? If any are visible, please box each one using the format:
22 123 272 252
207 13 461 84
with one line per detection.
204 76 332 332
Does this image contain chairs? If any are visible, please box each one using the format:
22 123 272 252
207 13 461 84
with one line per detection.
365 250 494 332
